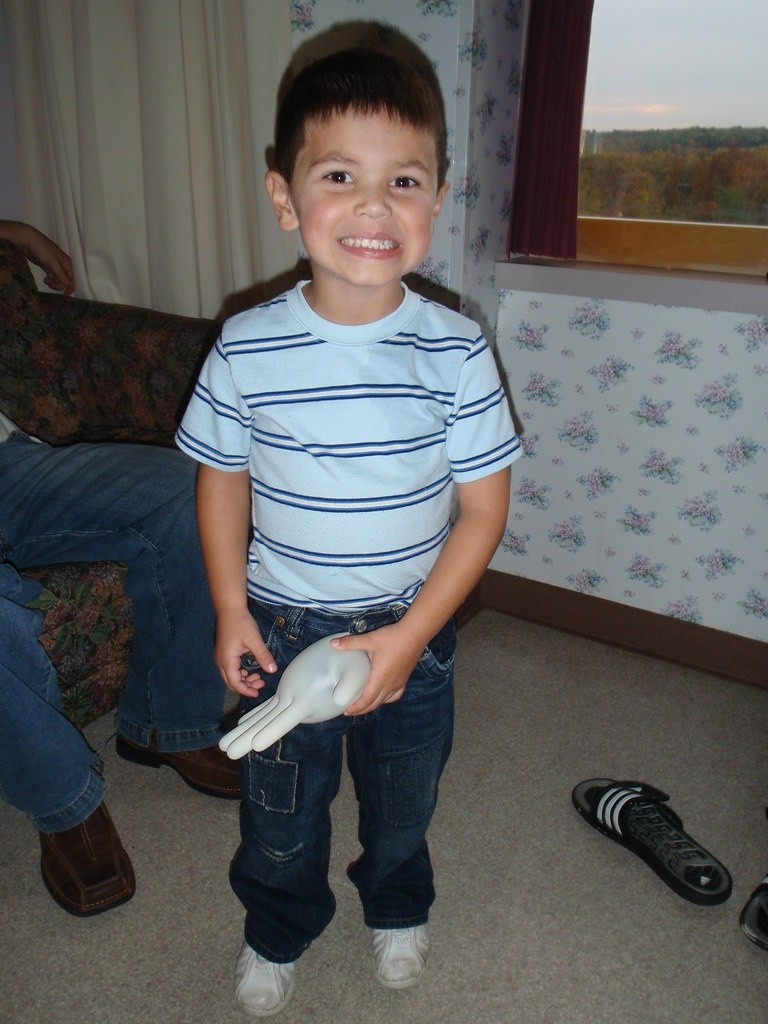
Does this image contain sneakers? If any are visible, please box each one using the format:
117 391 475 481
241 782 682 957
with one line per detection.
232 942 297 1017
373 925 430 992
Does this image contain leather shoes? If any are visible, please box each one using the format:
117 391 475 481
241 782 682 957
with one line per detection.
33 794 138 918
114 730 249 801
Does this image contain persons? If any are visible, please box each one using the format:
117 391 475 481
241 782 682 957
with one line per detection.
176 49 522 1016
0 222 252 924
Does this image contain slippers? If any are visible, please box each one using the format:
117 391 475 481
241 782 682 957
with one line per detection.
571 776 732 907
739 874 768 951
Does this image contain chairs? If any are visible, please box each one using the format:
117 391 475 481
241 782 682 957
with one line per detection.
0 238 227 736
